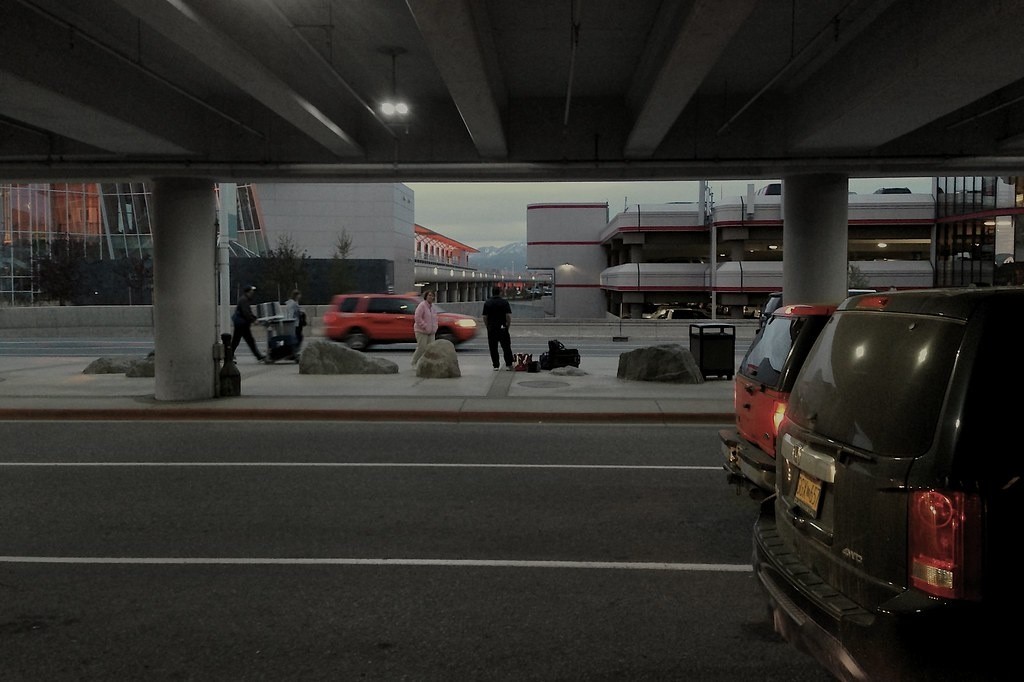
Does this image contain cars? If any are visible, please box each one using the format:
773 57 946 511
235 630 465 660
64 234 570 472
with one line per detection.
874 188 912 195
530 288 552 295
642 305 732 318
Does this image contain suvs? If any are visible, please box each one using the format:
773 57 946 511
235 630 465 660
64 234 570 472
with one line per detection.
753 183 781 196
756 292 782 334
750 287 1024 682
717 301 837 500
323 292 479 350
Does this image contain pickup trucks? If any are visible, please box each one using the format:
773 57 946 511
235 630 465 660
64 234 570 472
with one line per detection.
742 299 768 319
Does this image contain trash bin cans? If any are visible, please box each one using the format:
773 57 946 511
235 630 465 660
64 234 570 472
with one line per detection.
689 323 736 380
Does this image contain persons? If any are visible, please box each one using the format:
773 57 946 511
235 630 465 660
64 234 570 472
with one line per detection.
231 284 267 364
410 290 438 371
286 289 303 356
482 287 513 371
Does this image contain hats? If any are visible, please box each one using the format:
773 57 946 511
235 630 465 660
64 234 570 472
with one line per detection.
243 285 256 292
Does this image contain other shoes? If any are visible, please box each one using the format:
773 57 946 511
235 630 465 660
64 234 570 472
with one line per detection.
233 358 237 364
506 366 513 371
493 367 498 371
257 356 266 362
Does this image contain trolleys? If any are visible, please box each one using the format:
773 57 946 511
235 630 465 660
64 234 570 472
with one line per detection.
257 318 301 364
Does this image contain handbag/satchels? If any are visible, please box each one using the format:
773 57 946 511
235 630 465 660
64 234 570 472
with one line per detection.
527 361 541 372
548 340 564 353
